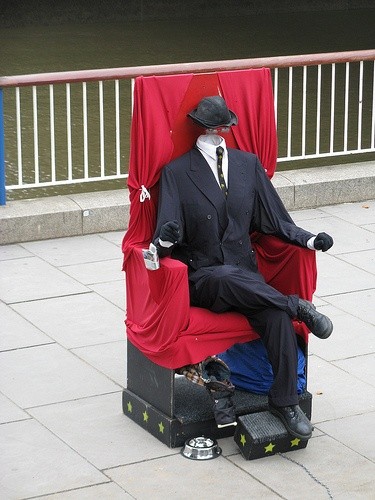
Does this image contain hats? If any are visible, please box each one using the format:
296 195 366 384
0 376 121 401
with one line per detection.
186 95 239 129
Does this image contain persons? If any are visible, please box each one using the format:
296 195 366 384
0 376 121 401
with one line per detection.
153 96 334 441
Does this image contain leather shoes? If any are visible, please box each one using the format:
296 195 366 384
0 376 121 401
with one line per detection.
268 403 313 441
297 298 333 339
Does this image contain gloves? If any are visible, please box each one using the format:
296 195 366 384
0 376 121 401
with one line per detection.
159 221 180 243
314 232 333 252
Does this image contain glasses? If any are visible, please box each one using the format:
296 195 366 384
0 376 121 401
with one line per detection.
201 127 230 135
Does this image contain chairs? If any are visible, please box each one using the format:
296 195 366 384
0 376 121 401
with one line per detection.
120 68 317 461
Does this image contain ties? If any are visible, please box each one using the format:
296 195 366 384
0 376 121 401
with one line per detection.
217 147 227 197
